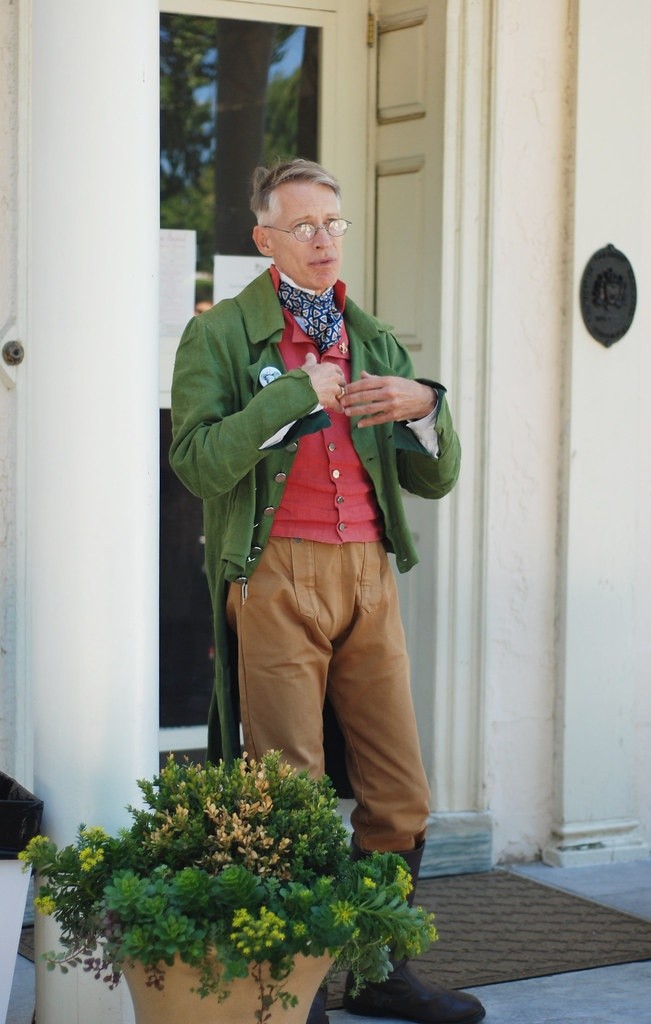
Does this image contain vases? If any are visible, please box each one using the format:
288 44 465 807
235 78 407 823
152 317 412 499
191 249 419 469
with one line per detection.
109 943 352 1024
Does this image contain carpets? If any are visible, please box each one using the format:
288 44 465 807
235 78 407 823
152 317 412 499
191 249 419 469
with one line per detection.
323 869 650 1012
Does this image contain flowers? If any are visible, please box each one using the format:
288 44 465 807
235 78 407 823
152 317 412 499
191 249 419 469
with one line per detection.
15 746 439 1023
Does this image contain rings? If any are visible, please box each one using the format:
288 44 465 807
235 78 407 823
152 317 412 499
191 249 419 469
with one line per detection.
337 386 345 400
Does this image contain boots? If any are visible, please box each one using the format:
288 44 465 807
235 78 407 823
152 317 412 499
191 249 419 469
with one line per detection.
344 832 486 1023
304 855 333 1023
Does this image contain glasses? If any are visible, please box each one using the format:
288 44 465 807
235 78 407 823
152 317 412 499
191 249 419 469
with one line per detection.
265 218 351 241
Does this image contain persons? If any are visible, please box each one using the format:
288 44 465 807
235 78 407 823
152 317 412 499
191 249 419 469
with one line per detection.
167 158 486 1024
